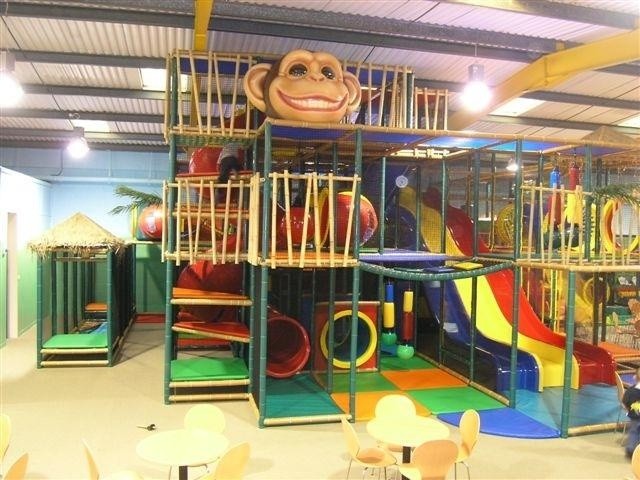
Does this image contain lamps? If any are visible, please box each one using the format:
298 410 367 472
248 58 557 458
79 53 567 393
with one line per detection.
506 154 519 171
0 50 26 108
459 44 492 113
68 118 90 159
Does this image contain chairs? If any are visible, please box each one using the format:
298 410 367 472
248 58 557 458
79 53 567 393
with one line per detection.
614 371 629 446
612 298 640 348
0 451 29 480
80 404 251 480
625 444 640 480
0 413 12 464
340 394 480 480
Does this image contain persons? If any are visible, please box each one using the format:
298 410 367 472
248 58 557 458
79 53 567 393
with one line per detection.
213 144 243 205
622 366 640 461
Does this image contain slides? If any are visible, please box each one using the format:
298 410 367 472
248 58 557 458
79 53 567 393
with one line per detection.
384 185 617 392
139 201 314 379
